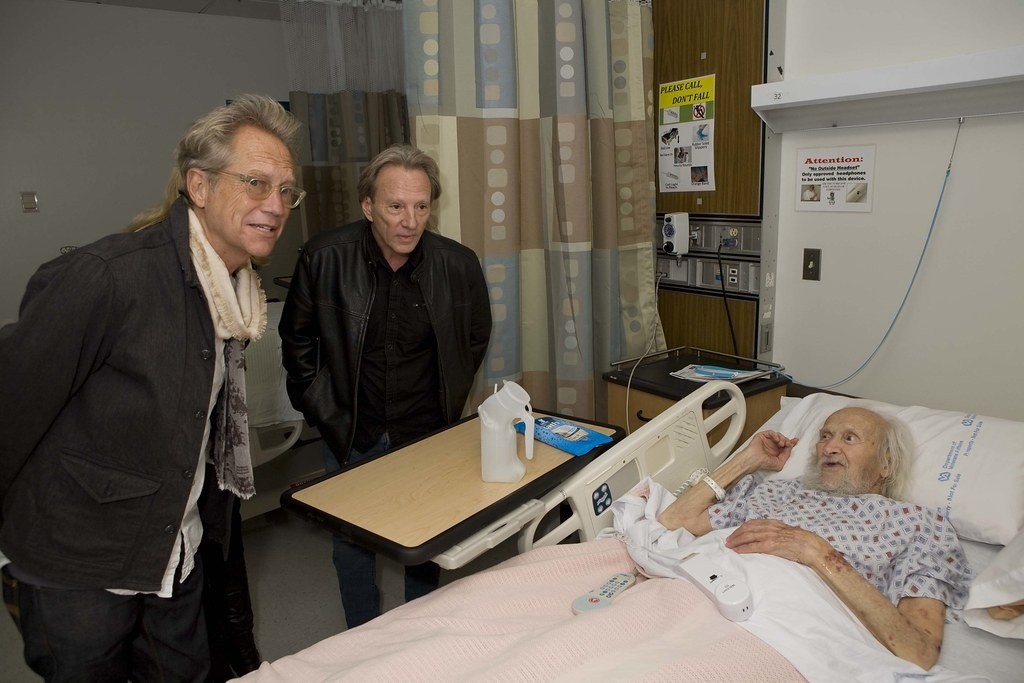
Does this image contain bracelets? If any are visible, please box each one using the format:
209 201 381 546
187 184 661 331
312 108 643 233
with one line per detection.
703 476 726 502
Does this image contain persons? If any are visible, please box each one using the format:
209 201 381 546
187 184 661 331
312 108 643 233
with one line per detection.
804 185 818 201
697 125 708 141
658 406 972 672
0 92 304 683
277 144 492 629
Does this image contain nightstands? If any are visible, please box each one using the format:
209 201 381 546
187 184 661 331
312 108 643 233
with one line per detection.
603 343 793 469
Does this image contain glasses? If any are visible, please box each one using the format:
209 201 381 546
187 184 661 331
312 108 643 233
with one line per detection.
206 168 307 209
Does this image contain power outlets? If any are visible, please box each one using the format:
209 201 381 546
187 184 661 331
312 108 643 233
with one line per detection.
720 226 744 252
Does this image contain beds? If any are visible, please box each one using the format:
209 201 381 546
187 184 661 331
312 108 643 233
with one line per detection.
226 376 1024 683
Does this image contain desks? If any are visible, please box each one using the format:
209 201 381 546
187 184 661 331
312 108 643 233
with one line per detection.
281 405 612 566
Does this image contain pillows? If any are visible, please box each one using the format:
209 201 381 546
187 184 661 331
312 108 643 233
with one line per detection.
752 393 1024 545
